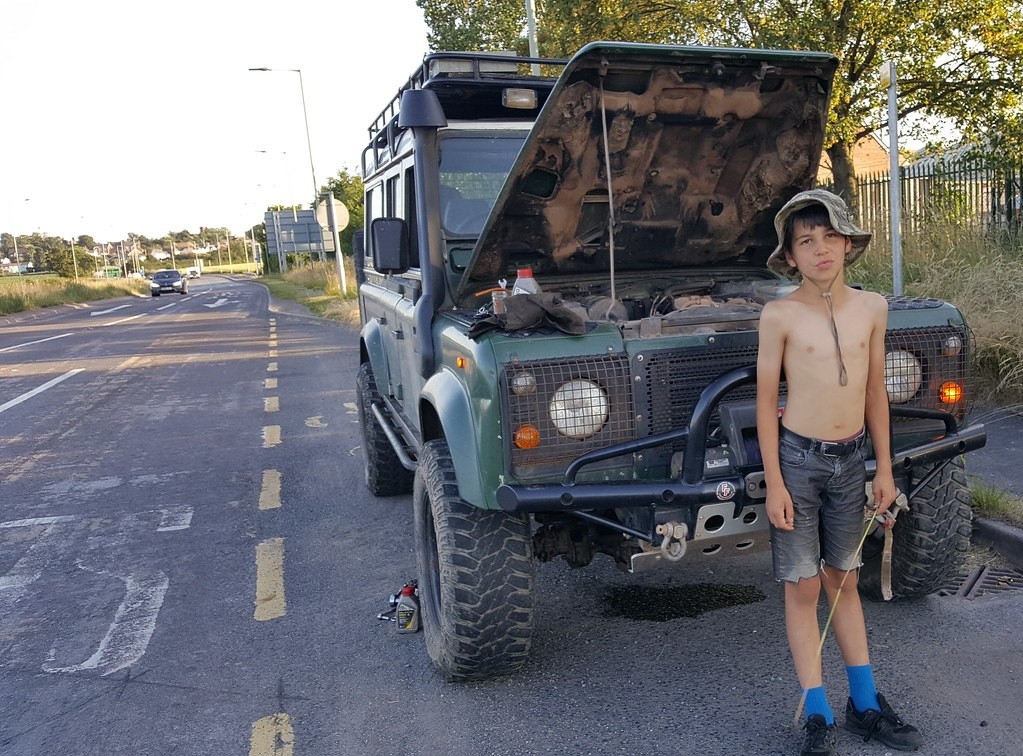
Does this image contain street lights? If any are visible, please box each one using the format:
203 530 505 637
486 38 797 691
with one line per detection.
248 67 327 262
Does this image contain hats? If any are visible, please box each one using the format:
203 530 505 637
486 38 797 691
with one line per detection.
767 189 873 282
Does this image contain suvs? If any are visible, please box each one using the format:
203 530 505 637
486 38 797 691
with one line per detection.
351 41 987 684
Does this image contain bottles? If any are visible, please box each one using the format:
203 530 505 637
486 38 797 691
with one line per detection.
511 269 541 296
396 588 418 634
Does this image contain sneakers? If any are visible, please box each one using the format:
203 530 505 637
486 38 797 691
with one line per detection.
798 713 839 756
843 693 924 750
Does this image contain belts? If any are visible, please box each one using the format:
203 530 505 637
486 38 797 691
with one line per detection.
778 417 868 458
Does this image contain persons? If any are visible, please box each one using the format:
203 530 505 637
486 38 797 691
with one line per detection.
755 189 926 756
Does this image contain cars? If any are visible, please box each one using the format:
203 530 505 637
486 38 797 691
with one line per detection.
189 270 200 279
148 274 154 279
150 270 188 297
92 266 122 277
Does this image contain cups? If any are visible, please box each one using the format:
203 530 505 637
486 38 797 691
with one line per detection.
492 290 512 315
